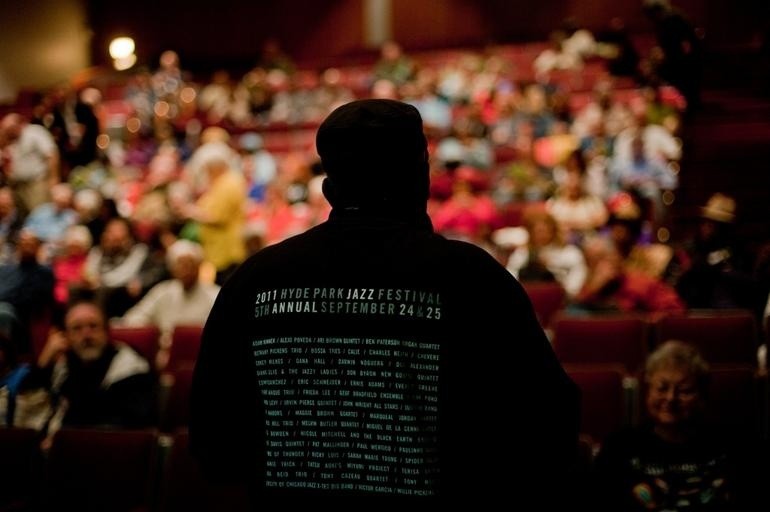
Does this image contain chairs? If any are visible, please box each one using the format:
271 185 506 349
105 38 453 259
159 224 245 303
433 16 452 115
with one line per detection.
2 273 770 510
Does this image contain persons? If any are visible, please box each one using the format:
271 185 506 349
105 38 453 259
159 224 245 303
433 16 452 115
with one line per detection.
1 21 770 512
